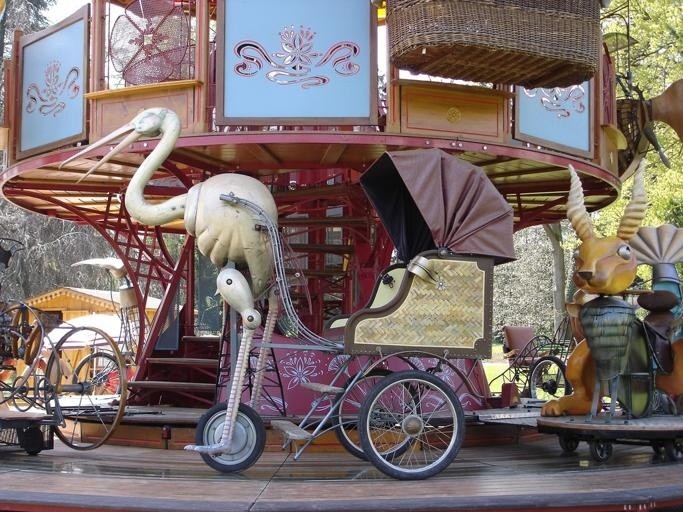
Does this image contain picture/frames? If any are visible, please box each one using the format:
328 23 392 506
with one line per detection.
513 76 594 159
215 0 379 126
15 4 91 160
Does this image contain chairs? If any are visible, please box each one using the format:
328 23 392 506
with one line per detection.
502 325 546 386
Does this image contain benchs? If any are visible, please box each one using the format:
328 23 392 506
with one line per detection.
323 249 493 359
